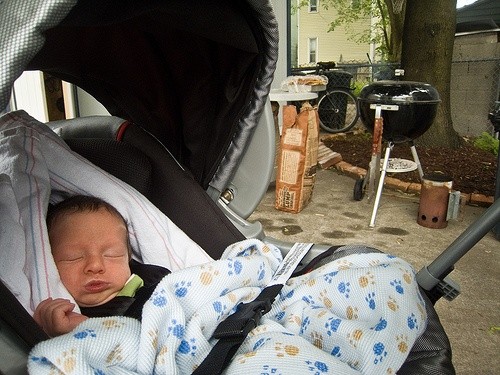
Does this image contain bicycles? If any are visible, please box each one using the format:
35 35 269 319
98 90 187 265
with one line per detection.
290 61 359 133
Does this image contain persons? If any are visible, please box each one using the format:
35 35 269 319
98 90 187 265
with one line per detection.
34 194 456 375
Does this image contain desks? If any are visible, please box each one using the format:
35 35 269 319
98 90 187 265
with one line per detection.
269 88 319 137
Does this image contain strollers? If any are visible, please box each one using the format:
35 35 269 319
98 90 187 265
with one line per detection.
0 0 499 375
